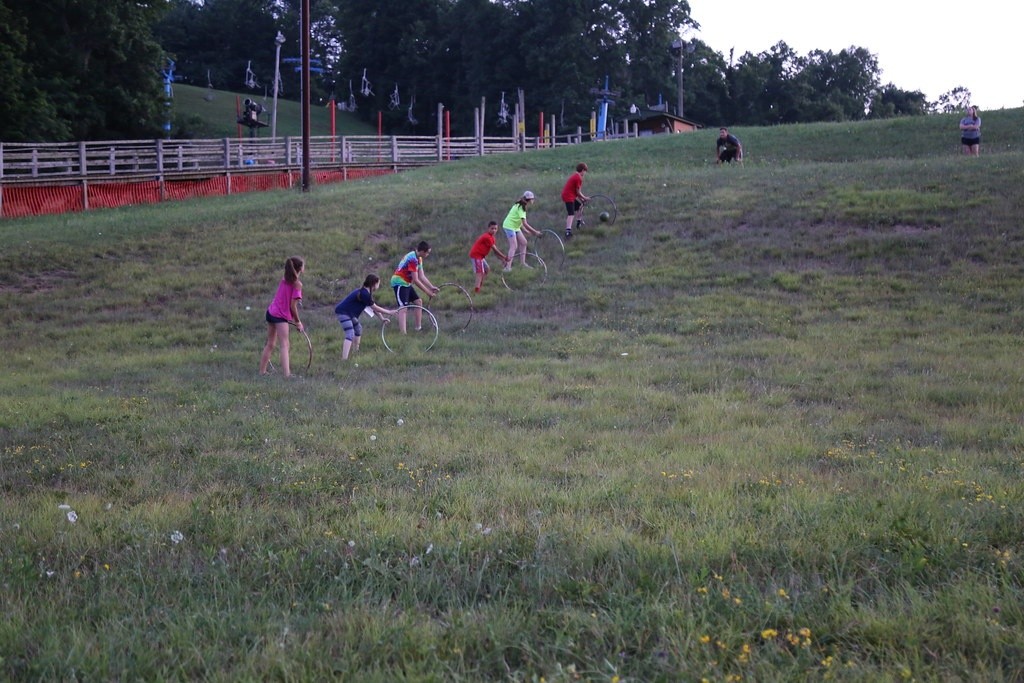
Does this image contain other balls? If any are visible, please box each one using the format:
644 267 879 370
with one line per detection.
598 211 611 223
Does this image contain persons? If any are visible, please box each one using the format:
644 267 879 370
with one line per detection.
959 107 981 156
256 257 305 379
715 128 742 164
469 221 510 292
502 191 542 272
335 274 399 361
391 241 439 335
561 163 589 238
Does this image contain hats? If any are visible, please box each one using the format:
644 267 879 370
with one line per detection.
523 191 535 199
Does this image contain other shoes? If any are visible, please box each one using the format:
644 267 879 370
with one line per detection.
566 231 573 239
504 268 512 272
576 219 585 229
520 263 533 270
